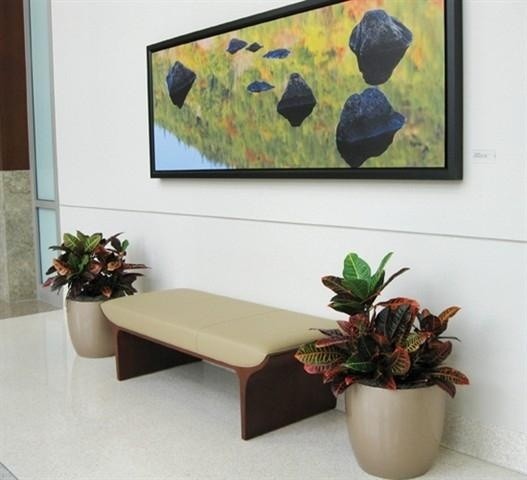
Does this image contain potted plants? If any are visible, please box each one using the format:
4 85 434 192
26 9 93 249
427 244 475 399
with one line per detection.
43 230 149 359
295 252 471 478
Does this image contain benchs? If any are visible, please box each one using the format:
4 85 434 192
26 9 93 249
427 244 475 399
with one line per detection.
100 287 346 440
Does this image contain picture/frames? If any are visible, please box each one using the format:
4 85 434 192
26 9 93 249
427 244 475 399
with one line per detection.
147 0 464 181
